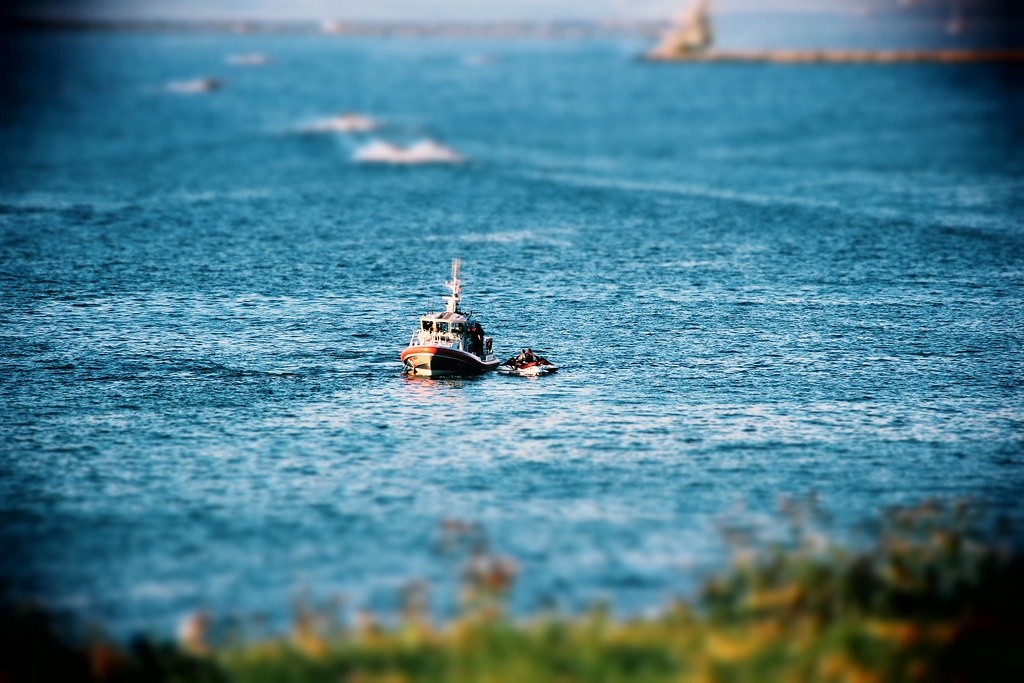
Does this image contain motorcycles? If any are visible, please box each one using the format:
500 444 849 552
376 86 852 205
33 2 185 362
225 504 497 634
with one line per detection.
497 355 558 377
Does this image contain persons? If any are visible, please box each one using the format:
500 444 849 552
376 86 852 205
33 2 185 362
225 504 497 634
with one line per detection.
525 349 534 363
517 349 526 363
470 323 493 357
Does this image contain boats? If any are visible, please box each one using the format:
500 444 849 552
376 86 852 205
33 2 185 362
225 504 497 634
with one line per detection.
400 257 501 378
